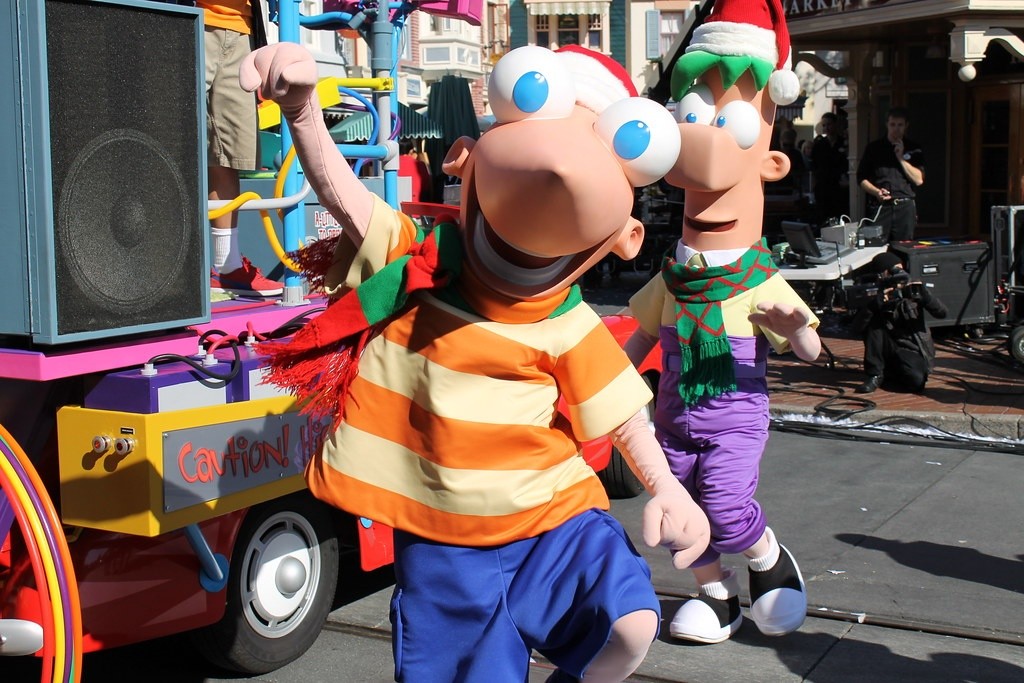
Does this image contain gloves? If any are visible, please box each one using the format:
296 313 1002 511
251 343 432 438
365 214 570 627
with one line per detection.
906 283 932 304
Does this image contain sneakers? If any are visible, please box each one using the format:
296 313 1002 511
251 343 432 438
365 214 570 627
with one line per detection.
210 253 285 296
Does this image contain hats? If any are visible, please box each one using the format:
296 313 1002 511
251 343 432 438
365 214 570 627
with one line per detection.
872 252 903 268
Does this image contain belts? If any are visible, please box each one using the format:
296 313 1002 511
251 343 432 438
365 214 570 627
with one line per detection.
883 198 913 206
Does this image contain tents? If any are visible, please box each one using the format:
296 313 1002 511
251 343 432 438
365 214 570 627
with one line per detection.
327 96 443 161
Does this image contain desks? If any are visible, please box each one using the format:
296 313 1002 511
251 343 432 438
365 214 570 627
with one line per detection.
769 240 893 375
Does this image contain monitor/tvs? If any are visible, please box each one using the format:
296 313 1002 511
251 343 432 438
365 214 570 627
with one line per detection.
781 221 821 269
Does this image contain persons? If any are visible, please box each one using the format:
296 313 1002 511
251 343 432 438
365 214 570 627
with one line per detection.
857 106 926 247
625 1 822 644
191 0 287 297
242 43 710 683
769 113 850 224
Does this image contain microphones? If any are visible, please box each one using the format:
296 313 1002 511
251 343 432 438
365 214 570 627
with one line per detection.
888 274 909 282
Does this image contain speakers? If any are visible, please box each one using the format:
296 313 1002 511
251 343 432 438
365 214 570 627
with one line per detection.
0 0 210 345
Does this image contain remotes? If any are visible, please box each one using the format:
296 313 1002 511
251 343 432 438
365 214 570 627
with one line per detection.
883 191 890 194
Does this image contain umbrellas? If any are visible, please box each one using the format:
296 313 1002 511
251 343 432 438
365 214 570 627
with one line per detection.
427 75 481 191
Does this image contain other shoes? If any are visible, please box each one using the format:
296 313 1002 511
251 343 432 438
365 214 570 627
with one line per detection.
856 375 886 392
